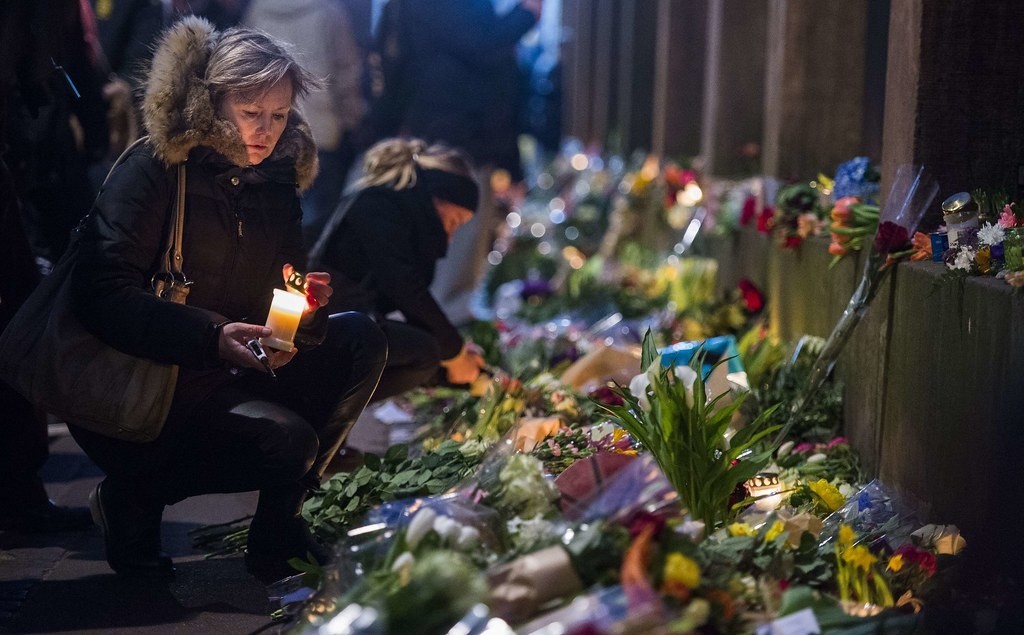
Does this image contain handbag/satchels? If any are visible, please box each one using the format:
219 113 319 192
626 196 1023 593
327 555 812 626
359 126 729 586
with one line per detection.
0 136 195 444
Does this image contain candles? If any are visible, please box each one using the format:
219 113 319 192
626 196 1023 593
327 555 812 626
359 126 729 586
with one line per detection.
257 286 303 354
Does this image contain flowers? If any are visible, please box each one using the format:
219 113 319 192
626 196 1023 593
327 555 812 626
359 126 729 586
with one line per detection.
189 137 1024 635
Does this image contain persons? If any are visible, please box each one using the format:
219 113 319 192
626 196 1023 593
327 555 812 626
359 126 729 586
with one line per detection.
32 11 388 579
0 0 540 551
306 134 485 472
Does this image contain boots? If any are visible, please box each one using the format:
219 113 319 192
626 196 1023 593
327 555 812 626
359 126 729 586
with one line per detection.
88 471 177 580
244 478 333 582
